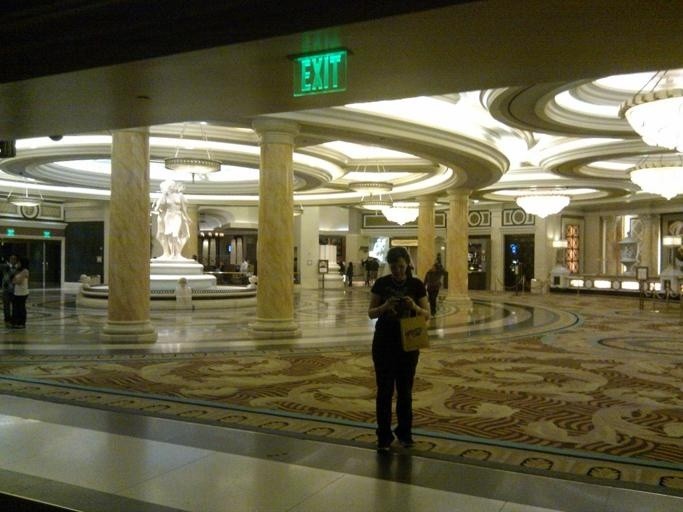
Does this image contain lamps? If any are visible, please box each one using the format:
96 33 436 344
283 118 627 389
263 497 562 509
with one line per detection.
515 193 572 219
348 157 420 226
618 69 683 200
165 121 221 174
7 176 44 206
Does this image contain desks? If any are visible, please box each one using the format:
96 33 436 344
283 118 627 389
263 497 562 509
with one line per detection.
203 272 248 285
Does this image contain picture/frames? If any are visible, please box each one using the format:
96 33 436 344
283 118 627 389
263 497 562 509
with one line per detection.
660 213 683 276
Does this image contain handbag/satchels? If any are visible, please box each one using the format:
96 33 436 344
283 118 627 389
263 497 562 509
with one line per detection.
399 306 429 352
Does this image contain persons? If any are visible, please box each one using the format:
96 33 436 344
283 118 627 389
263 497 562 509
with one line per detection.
0 254 31 329
362 257 379 286
337 260 354 287
425 262 450 319
365 245 431 453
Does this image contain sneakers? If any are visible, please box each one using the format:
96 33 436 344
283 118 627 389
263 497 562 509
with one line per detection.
397 436 415 446
375 436 390 452
